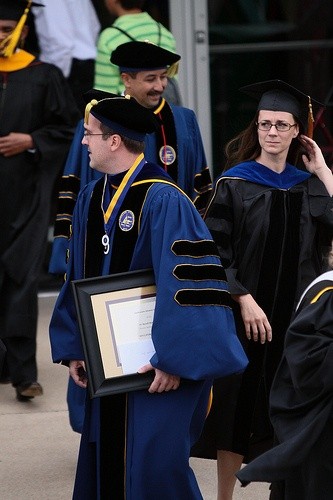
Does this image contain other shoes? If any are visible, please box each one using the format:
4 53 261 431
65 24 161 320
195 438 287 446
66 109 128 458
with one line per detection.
16 380 44 402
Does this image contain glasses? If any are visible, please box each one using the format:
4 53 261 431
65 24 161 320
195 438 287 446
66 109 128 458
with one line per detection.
254 119 297 132
83 130 124 141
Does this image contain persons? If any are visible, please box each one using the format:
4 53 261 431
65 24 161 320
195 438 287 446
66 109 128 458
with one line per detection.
191 80 333 500
48 95 248 500
0 0 213 402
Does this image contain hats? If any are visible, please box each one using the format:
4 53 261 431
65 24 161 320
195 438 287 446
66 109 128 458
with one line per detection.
0 0 46 59
234 77 326 140
109 40 181 72
80 88 162 142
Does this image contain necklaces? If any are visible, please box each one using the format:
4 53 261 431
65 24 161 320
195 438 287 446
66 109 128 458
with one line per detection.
100 153 145 255
